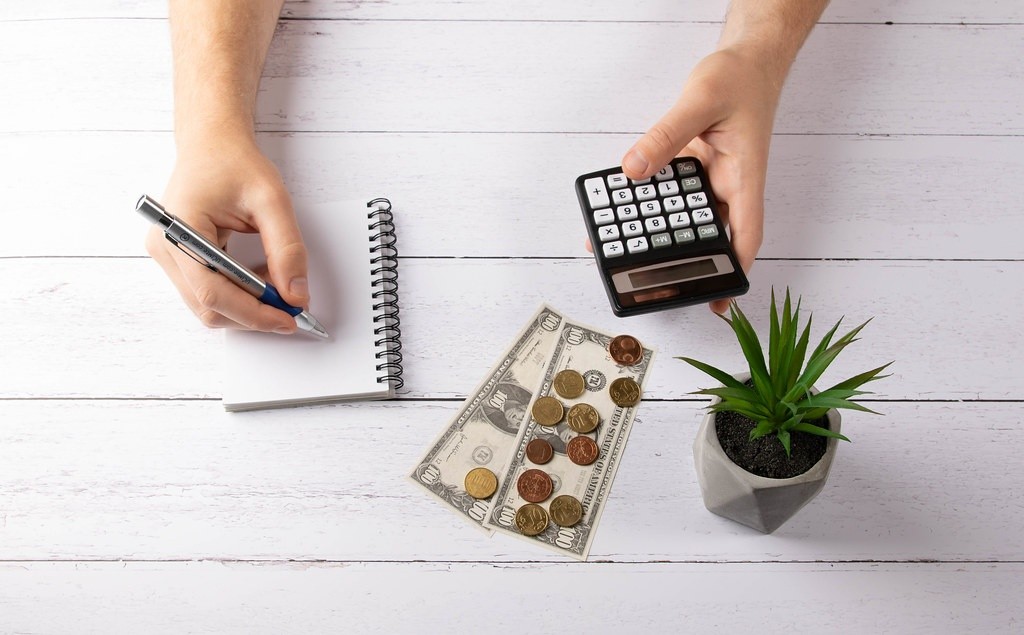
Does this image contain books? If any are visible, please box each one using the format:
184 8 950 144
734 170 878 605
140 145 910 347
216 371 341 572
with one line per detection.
220 196 405 413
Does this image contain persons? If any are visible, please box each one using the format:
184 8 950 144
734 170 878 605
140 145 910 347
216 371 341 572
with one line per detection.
147 0 831 335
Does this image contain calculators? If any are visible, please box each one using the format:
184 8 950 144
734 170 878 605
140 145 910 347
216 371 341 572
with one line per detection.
575 156 749 317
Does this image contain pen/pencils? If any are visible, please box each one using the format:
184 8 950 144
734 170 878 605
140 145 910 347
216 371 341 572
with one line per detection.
133 195 331 340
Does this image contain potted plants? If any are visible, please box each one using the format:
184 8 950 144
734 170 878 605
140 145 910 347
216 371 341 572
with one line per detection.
671 284 896 534
